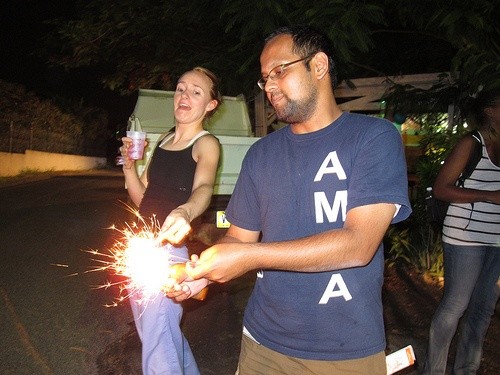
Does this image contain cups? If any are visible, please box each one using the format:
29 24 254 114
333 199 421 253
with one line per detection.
126 131 146 160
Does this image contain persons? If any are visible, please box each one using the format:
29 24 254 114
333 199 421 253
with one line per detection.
119 66 221 375
421 85 500 375
167 24 413 375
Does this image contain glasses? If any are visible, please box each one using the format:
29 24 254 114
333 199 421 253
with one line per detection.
257 51 318 91
